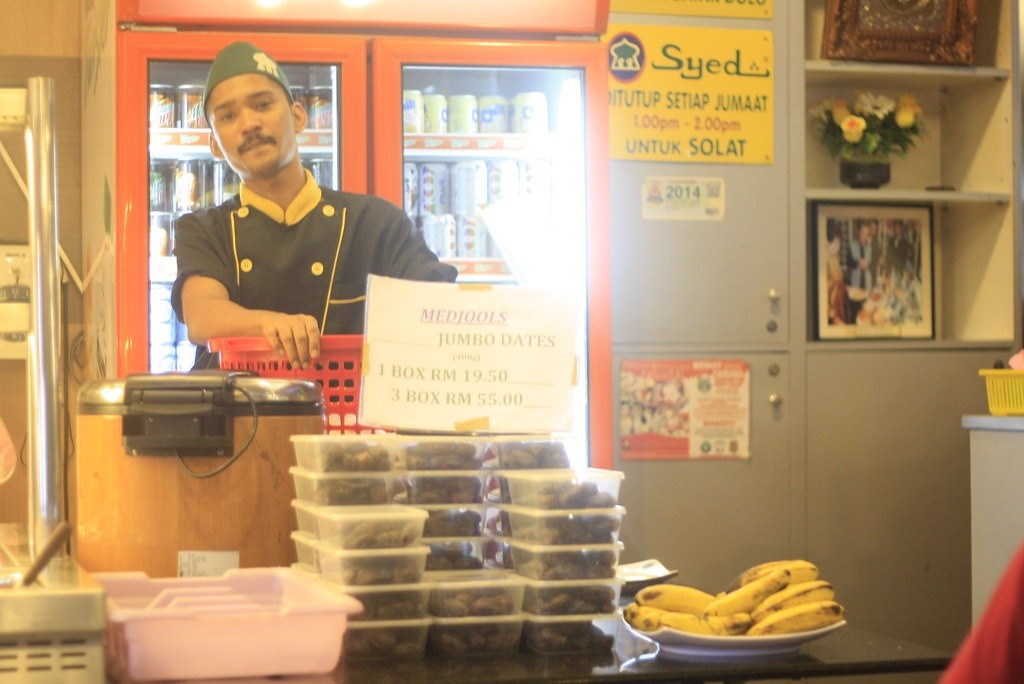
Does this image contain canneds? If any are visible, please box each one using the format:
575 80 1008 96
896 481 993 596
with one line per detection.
148 84 333 374
402 89 550 257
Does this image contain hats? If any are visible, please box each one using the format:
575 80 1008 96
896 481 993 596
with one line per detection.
202 41 293 134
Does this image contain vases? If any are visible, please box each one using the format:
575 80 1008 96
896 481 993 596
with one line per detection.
840 156 892 190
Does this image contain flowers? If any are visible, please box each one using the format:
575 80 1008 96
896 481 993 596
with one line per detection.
809 89 927 160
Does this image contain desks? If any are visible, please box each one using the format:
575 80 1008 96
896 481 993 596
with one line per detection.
134 585 953 684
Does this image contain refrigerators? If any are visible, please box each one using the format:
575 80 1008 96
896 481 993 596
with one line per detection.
74 0 616 581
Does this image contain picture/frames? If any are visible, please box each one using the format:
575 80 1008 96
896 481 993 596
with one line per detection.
820 1 978 66
809 198 936 340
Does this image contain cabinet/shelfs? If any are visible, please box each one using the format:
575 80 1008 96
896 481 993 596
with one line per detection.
805 45 1011 208
806 348 1014 669
614 351 798 595
607 0 791 344
959 412 1023 632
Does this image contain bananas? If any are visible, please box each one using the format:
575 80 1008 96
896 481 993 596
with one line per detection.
621 557 843 638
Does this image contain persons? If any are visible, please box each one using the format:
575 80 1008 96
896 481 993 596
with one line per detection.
171 42 461 371
825 221 873 326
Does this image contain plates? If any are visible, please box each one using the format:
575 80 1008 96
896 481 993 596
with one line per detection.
619 607 847 657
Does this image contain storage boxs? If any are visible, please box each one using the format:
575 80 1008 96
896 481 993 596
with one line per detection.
0 428 623 682
